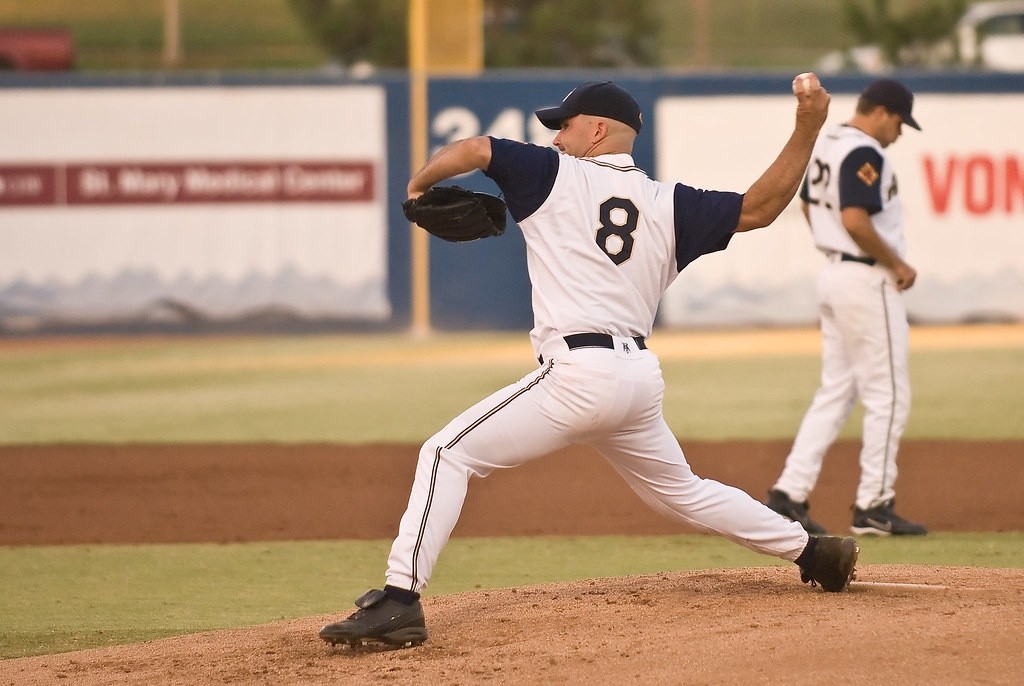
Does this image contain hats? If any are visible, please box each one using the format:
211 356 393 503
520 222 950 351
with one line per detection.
534 81 642 134
862 79 921 131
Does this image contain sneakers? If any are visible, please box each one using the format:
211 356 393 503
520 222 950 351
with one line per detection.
801 536 860 593
851 501 926 536
765 489 825 535
319 589 426 648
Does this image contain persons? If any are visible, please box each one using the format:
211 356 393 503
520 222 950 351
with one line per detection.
318 73 859 647
767 78 929 538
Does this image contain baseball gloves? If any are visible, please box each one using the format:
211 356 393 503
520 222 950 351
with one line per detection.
401 186 509 245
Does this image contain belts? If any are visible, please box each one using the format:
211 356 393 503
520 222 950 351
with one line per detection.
538 332 650 365
843 254 877 266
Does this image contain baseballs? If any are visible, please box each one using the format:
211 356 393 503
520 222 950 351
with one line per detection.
791 71 820 96
